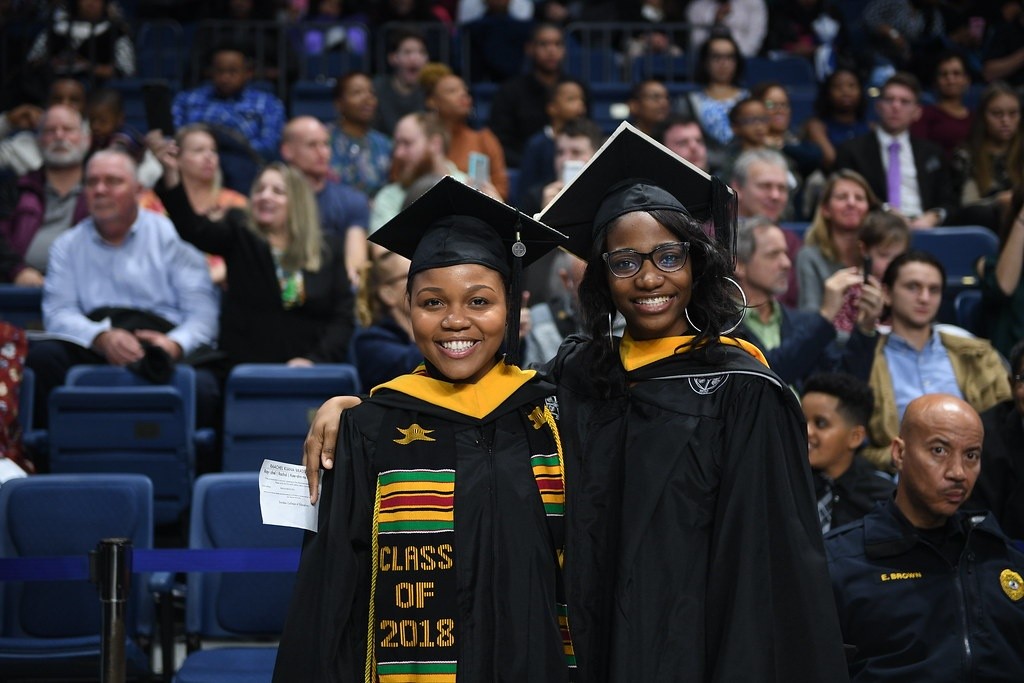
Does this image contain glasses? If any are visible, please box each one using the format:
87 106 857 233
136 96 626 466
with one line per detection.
601 242 691 278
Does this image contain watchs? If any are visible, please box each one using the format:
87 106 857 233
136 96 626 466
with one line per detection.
934 208 946 224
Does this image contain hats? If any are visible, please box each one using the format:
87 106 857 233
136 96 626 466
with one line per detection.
534 120 738 276
367 175 570 366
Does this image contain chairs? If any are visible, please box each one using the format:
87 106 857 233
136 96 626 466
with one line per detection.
782 220 1000 327
1 284 42 334
117 17 814 131
222 363 361 471
0 473 153 683
157 472 305 683
44 361 196 521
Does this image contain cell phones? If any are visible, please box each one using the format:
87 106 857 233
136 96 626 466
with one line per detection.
834 283 863 330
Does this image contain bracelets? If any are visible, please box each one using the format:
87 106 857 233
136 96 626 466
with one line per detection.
1017 216 1024 224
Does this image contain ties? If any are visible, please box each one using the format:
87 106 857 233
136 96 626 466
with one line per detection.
816 486 833 537
888 143 901 205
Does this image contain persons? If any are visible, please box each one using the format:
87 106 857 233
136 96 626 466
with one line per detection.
838 72 982 233
723 215 884 405
0 0 1024 683
153 123 260 264
861 249 1011 476
270 177 580 683
302 117 870 683
217 156 361 373
27 145 224 469
803 375 895 536
